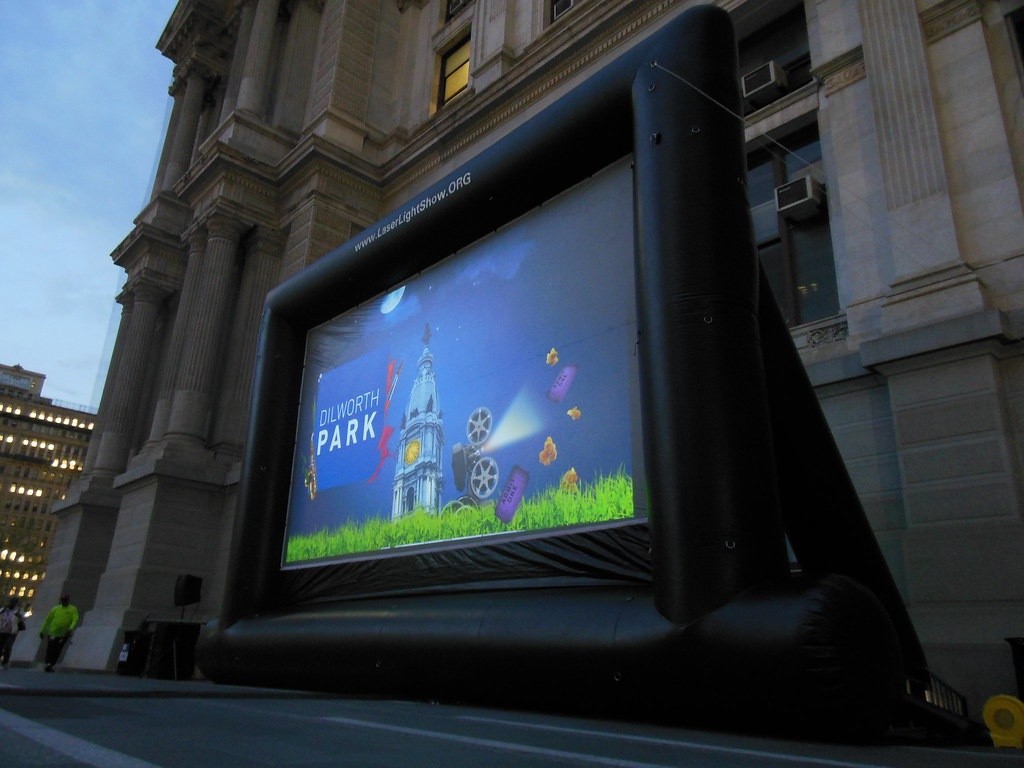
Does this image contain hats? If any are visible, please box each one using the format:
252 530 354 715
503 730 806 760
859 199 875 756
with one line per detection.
59 594 69 600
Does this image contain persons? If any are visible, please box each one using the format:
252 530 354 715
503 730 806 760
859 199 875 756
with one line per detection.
0 595 32 671
39 591 79 673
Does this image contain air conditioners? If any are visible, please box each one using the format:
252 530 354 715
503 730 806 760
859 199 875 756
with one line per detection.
773 176 827 222
741 60 790 104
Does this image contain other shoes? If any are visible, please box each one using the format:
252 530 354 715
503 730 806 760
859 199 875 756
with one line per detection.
39 633 44 639
1 660 7 665
66 630 72 637
44 665 55 673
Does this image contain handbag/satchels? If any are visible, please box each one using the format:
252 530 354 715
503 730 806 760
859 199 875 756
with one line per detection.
18 620 26 631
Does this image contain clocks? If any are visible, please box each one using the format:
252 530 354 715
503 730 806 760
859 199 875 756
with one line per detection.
404 439 421 465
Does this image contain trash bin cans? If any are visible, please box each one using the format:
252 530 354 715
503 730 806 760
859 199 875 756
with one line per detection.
1005 637 1024 703
116 630 154 678
